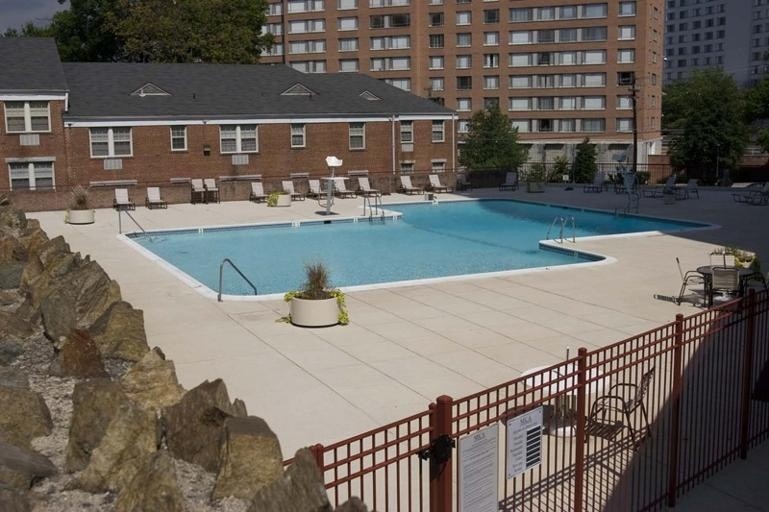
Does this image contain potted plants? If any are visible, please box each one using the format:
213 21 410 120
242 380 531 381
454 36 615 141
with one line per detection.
525 162 547 193
266 192 291 207
64 183 95 224
289 259 344 326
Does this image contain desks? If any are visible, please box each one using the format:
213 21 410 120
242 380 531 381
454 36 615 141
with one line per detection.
522 364 607 437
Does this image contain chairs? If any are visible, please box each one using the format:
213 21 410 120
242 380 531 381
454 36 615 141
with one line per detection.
113 188 135 210
249 181 268 203
399 174 472 195
306 178 381 200
145 186 167 210
583 172 700 200
582 367 658 454
675 252 768 313
728 182 769 206
190 178 220 205
499 172 520 192
282 180 302 201
